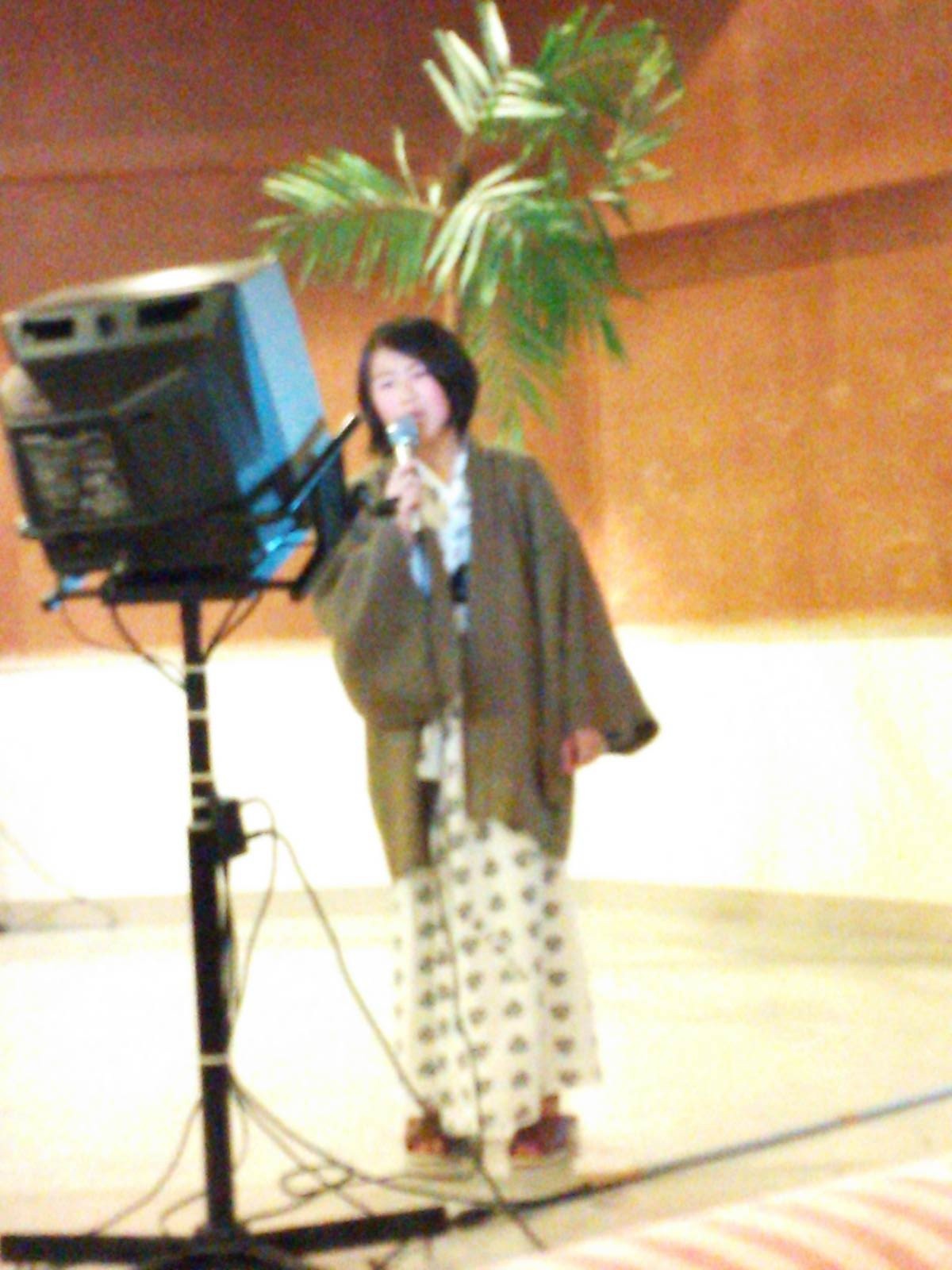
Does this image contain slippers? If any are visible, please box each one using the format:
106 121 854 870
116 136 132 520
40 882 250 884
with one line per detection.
405 1112 456 1165
511 1118 574 1171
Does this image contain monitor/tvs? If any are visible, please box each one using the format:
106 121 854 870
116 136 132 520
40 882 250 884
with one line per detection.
0 252 345 582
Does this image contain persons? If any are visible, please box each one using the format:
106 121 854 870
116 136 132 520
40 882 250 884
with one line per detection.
315 318 661 1162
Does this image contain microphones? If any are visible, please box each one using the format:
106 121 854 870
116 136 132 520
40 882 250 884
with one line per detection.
386 418 424 537
